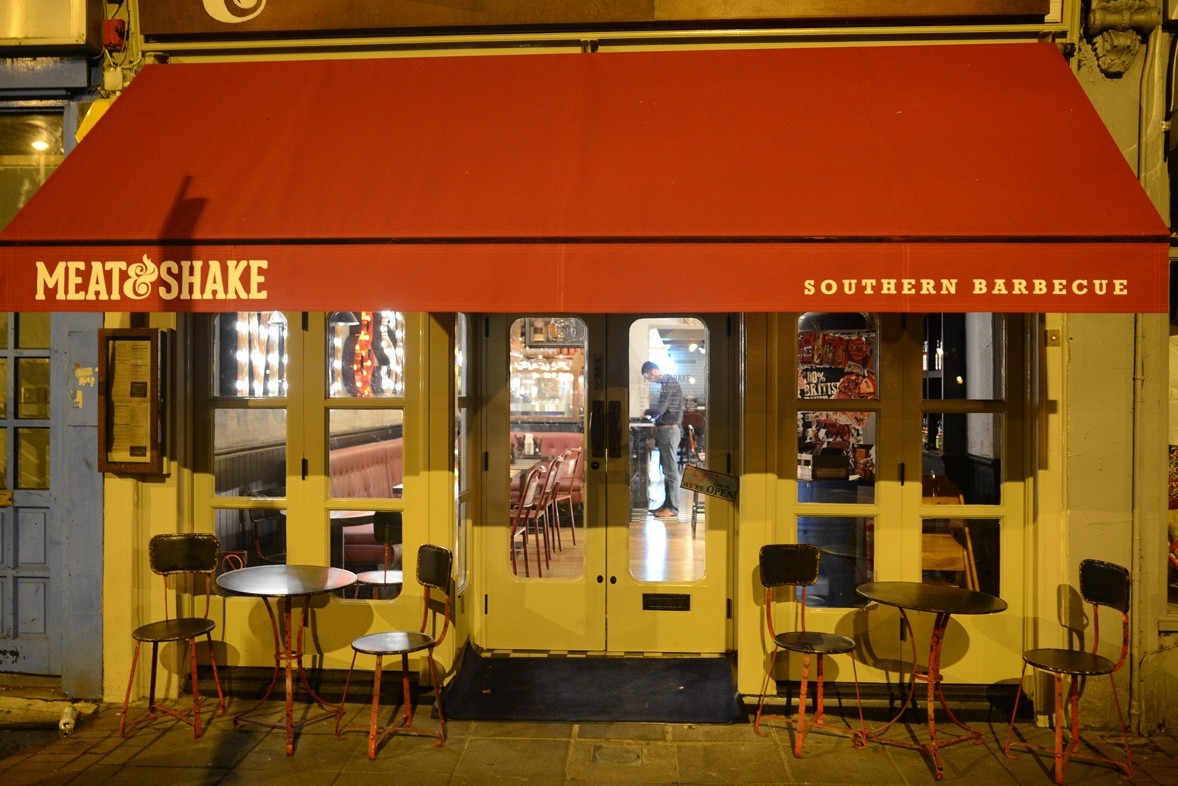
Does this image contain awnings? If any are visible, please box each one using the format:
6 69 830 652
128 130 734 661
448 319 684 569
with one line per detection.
0 42 1173 314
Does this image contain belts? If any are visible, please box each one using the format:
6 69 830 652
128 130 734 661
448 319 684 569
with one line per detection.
660 425 677 428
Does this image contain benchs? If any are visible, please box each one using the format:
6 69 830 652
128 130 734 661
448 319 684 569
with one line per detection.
331 436 402 498
510 430 586 521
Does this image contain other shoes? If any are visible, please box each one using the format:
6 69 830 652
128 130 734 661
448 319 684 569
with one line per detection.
651 506 678 517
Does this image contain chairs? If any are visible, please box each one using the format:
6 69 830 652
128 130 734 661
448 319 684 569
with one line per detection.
243 482 286 558
861 473 983 593
510 468 542 578
750 542 870 761
510 458 560 578
535 481 560 561
332 540 461 765
1002 558 1135 784
554 448 581 551
354 512 403 602
687 423 706 468
115 531 227 744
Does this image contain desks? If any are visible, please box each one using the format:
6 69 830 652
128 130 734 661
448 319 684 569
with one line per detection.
275 504 374 600
510 470 522 483
212 562 358 757
852 577 1009 780
512 455 542 500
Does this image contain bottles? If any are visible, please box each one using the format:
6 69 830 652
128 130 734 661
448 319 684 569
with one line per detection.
524 433 535 455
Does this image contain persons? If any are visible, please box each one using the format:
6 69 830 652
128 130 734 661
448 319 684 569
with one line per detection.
639 361 683 517
